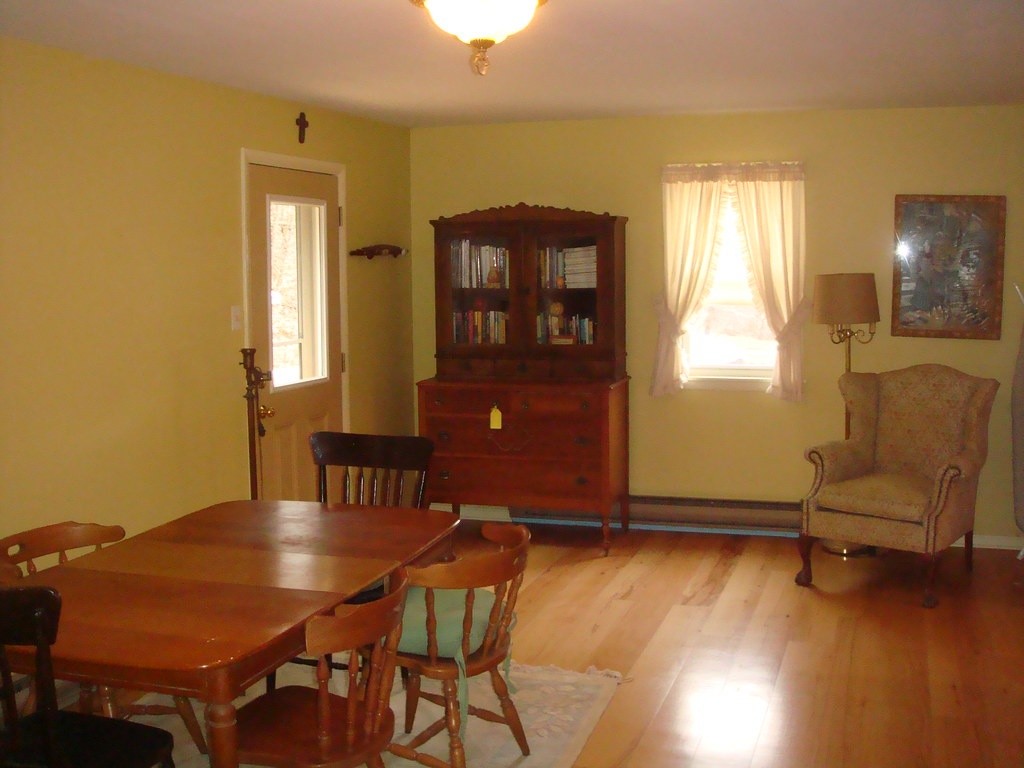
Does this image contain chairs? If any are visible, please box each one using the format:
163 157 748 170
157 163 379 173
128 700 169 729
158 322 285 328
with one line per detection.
0 521 209 755
358 522 532 768
267 431 435 695
236 566 410 768
0 585 174 768
795 364 1001 607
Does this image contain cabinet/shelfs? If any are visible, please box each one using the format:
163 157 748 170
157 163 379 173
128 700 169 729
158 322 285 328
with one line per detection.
415 202 635 558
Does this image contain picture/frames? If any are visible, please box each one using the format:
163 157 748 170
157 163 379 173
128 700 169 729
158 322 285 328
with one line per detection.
891 194 1007 340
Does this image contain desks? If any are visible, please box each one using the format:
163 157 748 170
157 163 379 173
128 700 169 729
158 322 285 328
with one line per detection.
0 499 461 768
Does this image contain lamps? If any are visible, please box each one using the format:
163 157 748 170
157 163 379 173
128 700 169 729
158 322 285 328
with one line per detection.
409 0 548 76
811 272 880 555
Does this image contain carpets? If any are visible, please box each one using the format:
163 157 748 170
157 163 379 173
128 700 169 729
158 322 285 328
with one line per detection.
127 651 623 768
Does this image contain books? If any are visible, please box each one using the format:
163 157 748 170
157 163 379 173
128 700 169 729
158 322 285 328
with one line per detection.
454 310 593 346
451 238 597 289
